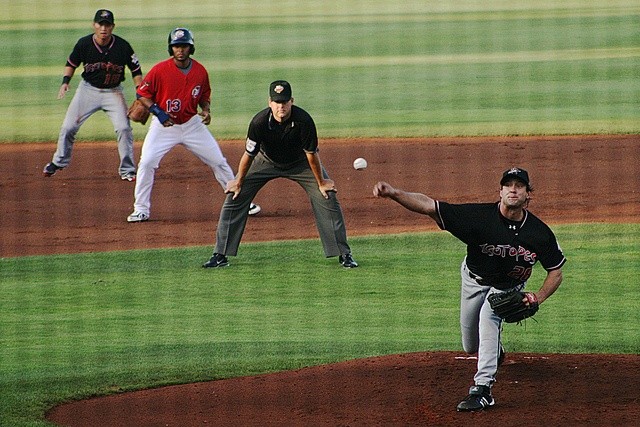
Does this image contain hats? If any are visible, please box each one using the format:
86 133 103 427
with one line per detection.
270 80 292 101
94 9 114 24
500 167 530 185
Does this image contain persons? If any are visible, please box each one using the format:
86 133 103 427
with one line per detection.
127 29 262 223
202 80 359 267
43 10 150 182
373 167 567 412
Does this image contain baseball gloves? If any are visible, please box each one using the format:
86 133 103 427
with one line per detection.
128 100 150 126
487 288 540 332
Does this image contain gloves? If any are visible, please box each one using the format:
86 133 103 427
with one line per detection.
149 103 171 124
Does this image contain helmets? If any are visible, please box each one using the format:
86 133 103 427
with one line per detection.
167 28 195 56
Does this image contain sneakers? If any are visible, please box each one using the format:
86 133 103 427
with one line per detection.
42 161 68 176
202 253 230 268
489 343 505 385
121 172 136 181
127 210 149 222
457 385 495 412
248 203 261 215
339 252 358 268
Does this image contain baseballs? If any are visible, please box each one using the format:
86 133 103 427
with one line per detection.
353 157 368 171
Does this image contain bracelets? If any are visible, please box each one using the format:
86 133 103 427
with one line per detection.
62 76 71 84
